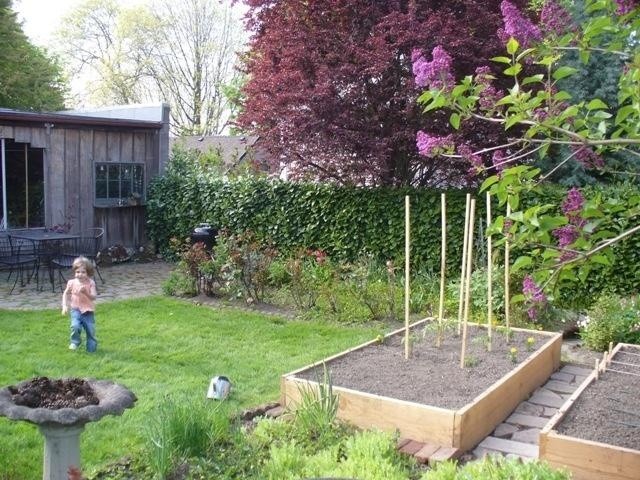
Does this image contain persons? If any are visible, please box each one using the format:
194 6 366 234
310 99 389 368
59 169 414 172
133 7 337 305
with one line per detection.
61 254 98 354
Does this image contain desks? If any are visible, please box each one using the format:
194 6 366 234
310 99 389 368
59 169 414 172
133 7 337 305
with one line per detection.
12 232 81 291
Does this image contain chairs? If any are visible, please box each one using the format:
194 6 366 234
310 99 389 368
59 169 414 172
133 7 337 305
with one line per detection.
48 227 105 295
0 236 39 295
